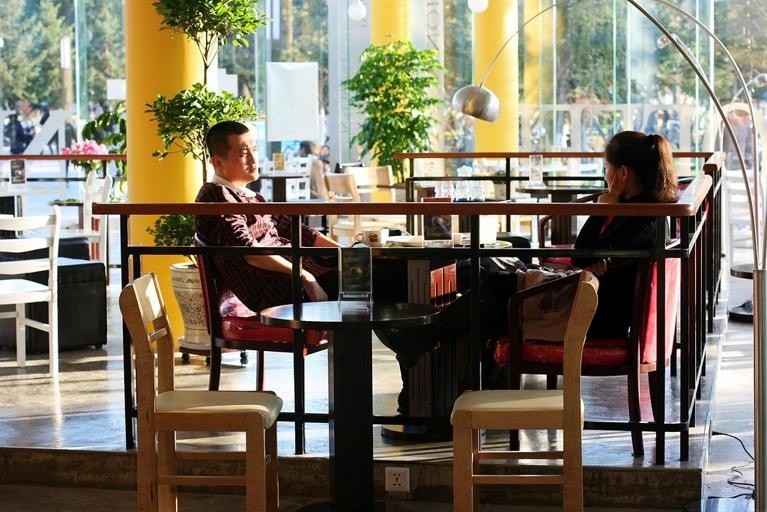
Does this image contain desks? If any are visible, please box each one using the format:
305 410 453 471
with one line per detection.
259 168 310 201
320 233 532 445
514 183 611 243
260 299 442 462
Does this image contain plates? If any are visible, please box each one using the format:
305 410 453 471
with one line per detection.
425 240 453 248
388 236 422 246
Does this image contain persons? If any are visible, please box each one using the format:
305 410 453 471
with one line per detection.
297 141 312 157
194 120 408 415
373 131 680 392
93 100 108 119
319 145 329 163
15 100 42 134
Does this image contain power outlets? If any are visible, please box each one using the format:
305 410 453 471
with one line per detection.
384 466 411 492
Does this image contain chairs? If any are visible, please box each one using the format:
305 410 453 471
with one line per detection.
32 170 114 286
323 172 396 235
192 230 335 457
368 163 410 228
489 239 681 465
1 183 62 380
118 272 282 511
451 268 601 512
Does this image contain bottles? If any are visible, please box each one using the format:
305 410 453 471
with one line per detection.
423 166 486 202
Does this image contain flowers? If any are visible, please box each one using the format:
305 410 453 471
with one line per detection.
60 138 106 176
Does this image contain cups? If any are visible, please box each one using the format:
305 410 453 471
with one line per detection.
529 155 544 186
355 228 389 248
452 233 463 244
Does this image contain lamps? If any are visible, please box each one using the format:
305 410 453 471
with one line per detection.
451 0 766 512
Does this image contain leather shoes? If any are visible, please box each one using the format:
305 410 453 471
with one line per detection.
373 328 418 368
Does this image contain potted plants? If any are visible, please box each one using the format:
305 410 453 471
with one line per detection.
142 0 267 353
